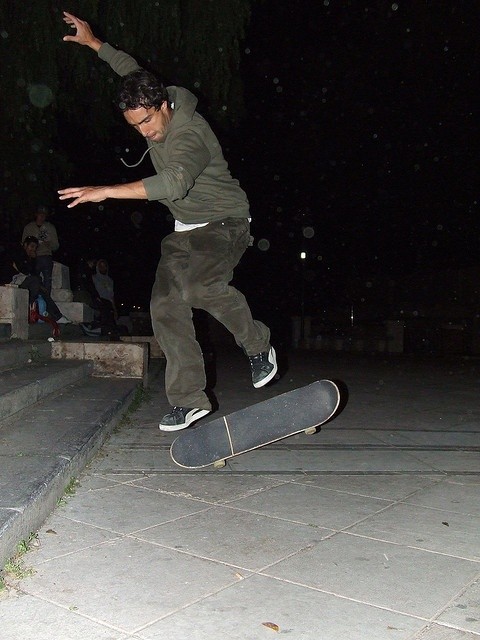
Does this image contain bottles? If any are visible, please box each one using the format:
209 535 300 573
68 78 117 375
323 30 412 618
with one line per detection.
34 294 47 322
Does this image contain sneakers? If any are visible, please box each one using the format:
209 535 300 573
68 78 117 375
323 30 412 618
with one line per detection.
249 345 278 389
158 406 211 431
57 316 71 324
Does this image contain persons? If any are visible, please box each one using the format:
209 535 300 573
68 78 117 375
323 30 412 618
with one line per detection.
71 257 119 338
56 11 278 432
10 236 72 324
23 205 61 294
92 253 122 341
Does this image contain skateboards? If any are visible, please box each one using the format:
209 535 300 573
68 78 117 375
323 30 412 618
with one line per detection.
170 379 341 470
